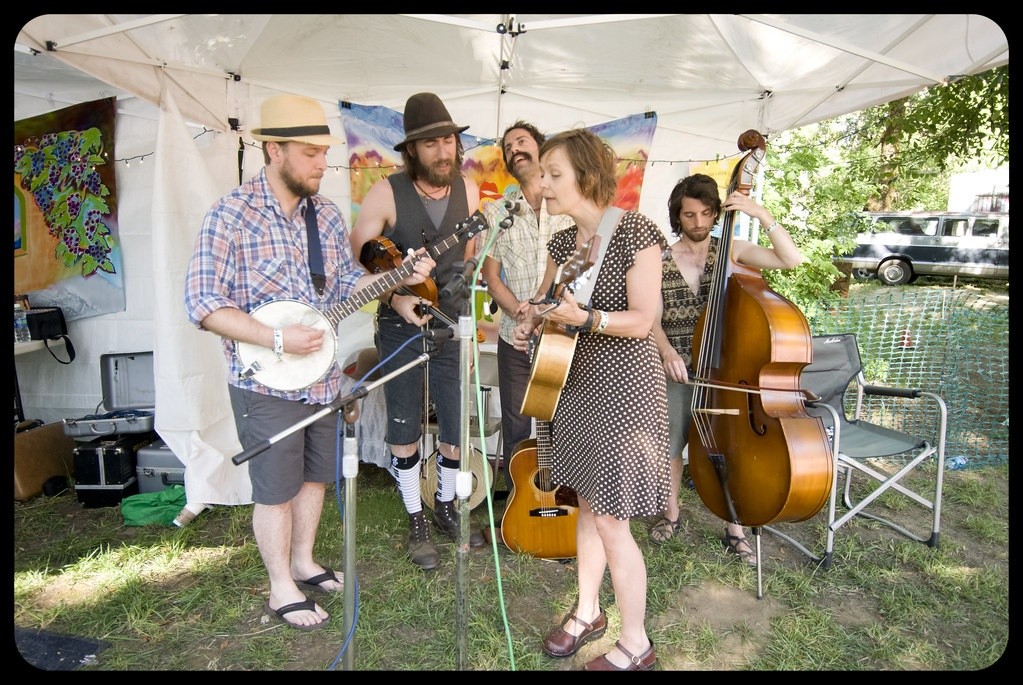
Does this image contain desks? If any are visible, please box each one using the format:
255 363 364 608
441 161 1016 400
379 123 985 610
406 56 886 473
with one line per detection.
14 335 69 429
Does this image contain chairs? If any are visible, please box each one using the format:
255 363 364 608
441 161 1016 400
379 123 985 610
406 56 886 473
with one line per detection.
419 351 503 510
761 333 947 572
899 221 925 235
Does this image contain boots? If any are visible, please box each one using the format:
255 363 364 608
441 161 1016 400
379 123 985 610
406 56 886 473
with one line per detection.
408 503 439 568
432 492 485 546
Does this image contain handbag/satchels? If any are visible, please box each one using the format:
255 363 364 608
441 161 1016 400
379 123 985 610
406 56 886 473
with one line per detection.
26 306 76 364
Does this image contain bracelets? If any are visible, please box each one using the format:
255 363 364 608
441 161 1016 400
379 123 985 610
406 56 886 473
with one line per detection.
763 219 780 236
566 303 609 333
387 292 395 308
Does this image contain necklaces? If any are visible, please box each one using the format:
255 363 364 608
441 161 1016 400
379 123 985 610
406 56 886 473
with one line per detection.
273 328 284 365
414 177 449 201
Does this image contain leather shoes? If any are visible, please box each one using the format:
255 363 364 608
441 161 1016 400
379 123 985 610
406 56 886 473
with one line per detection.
544 605 607 658
583 638 656 671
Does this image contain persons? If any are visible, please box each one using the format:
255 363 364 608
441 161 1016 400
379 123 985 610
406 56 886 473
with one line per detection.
513 122 672 671
474 116 577 544
350 93 488 569
185 92 437 630
647 172 803 566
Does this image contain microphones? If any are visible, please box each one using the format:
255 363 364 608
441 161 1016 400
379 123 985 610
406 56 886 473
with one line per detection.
422 324 460 341
505 199 527 215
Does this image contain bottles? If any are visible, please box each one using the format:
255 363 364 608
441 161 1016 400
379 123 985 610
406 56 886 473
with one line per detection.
949 454 968 471
14 303 31 343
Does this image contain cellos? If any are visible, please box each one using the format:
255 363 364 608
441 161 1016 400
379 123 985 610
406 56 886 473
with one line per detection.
687 126 836 600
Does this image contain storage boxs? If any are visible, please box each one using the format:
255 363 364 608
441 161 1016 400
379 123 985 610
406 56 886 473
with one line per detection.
14 420 76 501
72 432 160 508
136 439 187 493
61 350 154 436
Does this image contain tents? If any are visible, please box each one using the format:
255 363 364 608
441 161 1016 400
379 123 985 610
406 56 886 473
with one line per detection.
15 14 1009 244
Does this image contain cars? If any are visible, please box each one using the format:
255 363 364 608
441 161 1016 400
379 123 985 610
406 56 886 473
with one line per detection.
830 210 1009 288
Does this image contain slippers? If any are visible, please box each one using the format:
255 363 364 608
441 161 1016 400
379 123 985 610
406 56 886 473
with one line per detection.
265 594 331 631
293 566 340 596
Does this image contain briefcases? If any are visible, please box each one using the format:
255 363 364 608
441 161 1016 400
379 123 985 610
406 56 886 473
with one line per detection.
14 418 75 501
62 350 154 436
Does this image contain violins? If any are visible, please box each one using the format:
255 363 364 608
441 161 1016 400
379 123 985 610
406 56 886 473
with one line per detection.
359 234 487 344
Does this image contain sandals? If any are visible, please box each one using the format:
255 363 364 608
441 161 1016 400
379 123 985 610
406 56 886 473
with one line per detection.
648 515 680 547
721 528 757 566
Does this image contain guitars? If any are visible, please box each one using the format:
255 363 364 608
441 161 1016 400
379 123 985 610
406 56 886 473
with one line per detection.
235 208 491 394
498 333 581 561
517 232 603 423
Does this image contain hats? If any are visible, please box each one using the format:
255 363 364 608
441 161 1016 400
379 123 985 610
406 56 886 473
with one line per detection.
344 347 381 381
394 93 469 152
250 95 346 146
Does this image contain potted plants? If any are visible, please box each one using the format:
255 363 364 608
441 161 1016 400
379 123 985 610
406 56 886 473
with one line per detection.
805 172 890 316
